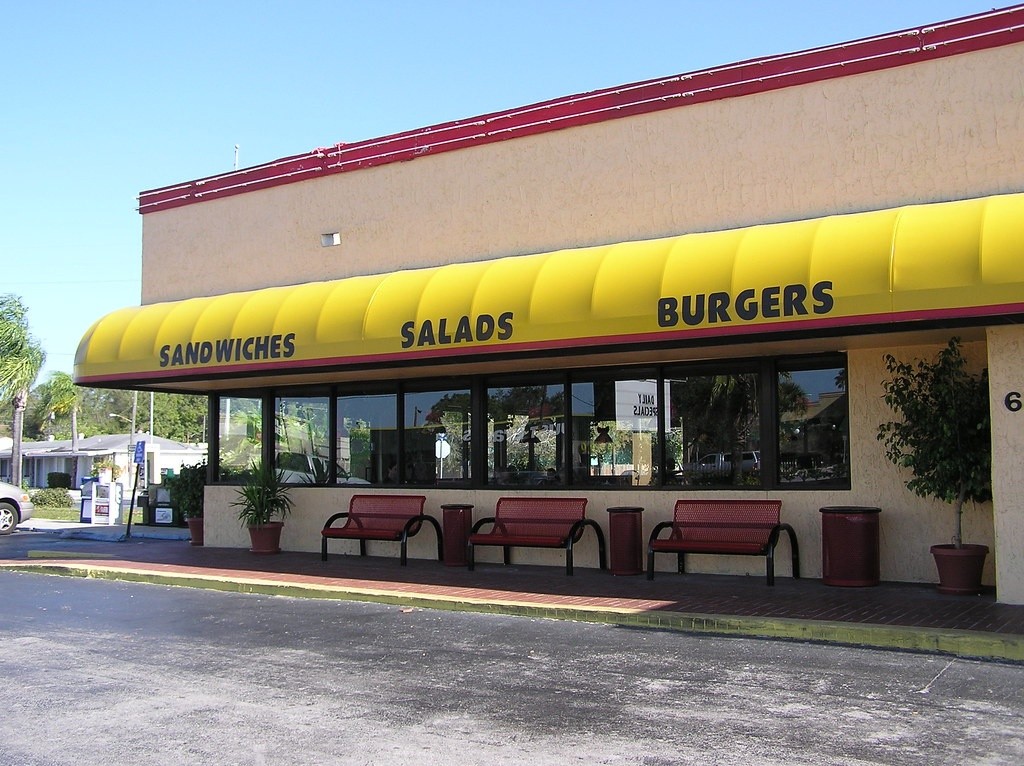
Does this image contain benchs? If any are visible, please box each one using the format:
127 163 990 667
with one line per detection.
320 494 446 567
643 499 805 588
464 496 608 577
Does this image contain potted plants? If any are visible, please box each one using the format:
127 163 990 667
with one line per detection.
228 452 296 554
873 335 994 594
159 457 230 547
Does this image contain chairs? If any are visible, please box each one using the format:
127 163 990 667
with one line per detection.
619 470 640 486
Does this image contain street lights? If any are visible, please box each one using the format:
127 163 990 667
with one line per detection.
109 413 135 488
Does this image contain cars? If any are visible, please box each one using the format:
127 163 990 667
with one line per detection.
0 480 35 535
275 450 372 485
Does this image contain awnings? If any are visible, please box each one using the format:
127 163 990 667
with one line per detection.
73 188 1024 392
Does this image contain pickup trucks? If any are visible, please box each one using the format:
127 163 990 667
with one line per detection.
695 451 761 474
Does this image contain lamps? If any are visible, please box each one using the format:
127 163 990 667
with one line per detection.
593 421 614 444
519 431 541 444
